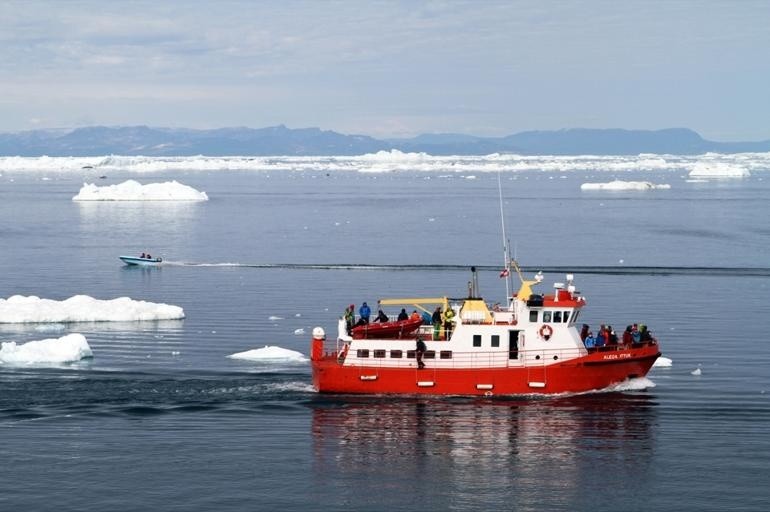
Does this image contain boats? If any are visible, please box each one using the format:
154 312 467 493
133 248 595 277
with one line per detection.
310 168 662 396
119 255 162 266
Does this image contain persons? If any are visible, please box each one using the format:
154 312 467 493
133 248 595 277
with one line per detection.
421 305 456 342
554 311 568 323
415 337 427 368
147 254 151 259
397 308 420 322
579 322 652 352
140 252 145 258
343 301 389 337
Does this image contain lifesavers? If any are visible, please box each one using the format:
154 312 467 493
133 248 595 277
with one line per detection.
540 325 553 339
